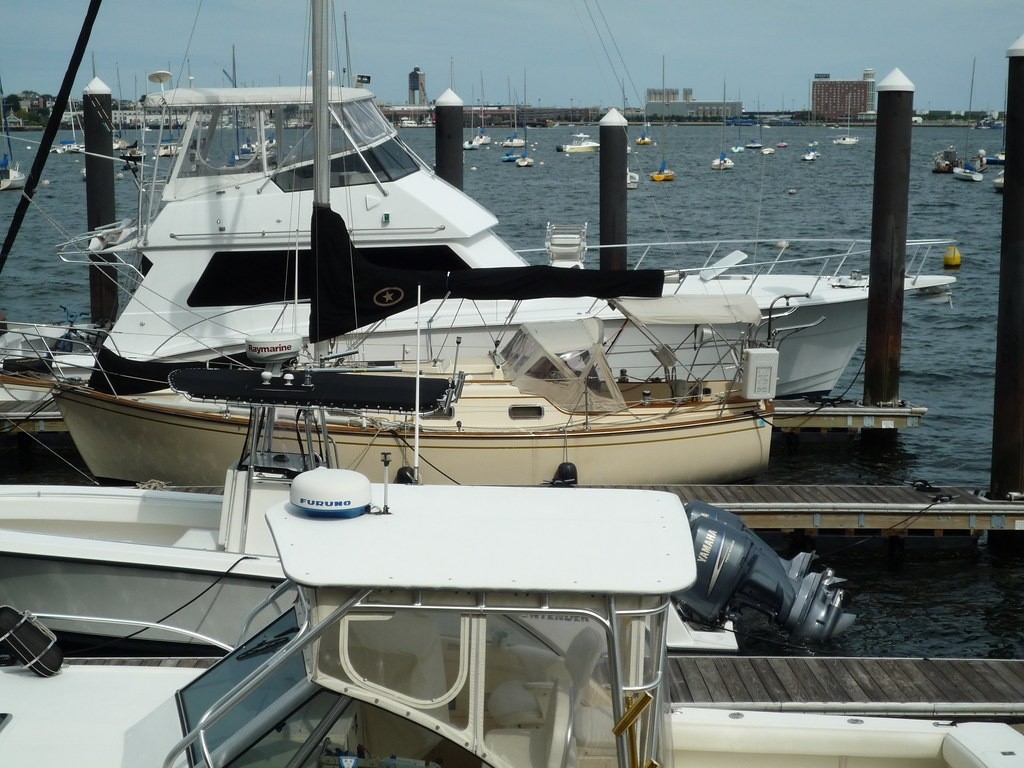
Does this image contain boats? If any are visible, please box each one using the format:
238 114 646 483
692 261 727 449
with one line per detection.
1 467 1024 768
62 1 775 490
59 78 961 401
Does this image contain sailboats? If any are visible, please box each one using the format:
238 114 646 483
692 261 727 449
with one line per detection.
0 44 298 196
398 56 1004 191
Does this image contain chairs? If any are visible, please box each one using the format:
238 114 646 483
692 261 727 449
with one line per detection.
482 625 604 768
